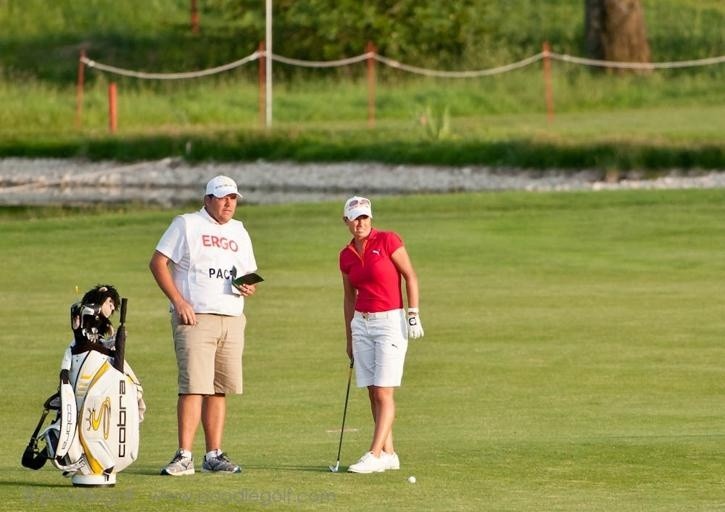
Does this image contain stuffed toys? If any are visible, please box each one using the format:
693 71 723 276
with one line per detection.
70 284 120 357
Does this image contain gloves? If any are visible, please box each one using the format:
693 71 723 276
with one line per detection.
406 307 424 338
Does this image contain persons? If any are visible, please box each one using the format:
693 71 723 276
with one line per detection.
340 195 424 475
149 174 257 477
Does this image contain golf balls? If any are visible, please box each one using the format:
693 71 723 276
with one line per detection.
407 477 416 484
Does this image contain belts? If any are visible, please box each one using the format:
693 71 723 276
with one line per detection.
353 310 405 321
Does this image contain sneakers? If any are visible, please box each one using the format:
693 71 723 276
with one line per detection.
346 449 400 474
201 453 242 474
160 454 195 476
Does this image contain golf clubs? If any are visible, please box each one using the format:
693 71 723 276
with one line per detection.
70 303 101 343
329 354 354 473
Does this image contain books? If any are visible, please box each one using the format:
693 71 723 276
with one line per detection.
228 272 265 295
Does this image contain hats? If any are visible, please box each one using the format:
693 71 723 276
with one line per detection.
343 195 373 222
206 175 244 199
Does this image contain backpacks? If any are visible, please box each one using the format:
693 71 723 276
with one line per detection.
21 339 146 488
